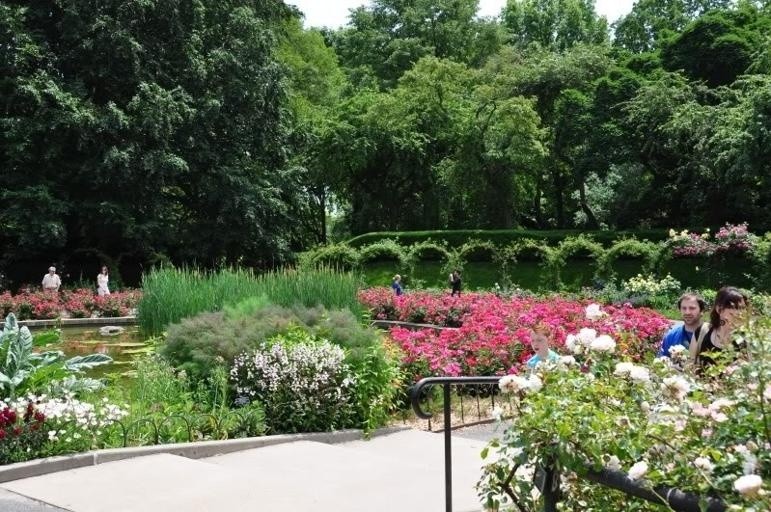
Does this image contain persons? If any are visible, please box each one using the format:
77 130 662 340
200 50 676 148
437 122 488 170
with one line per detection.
650 289 714 364
686 286 753 373
390 274 401 297
96 264 110 299
40 266 61 297
526 323 576 374
448 269 462 299
